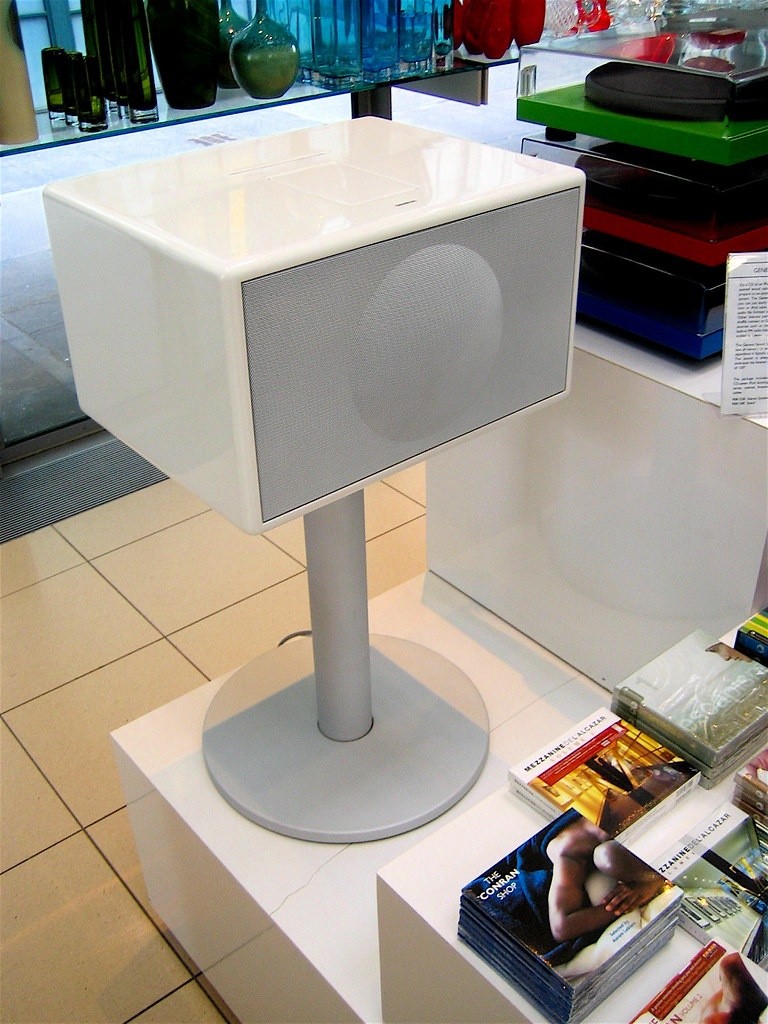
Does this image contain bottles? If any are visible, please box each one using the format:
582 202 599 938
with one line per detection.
450 0 610 59
147 0 219 110
80 0 158 124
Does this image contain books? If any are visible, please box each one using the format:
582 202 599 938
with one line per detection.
458 607 768 1023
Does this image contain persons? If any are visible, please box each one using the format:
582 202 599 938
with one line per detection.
546 815 669 944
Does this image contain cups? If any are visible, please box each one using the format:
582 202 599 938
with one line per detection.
72 56 108 132
265 0 455 91
41 47 64 118
56 52 93 126
